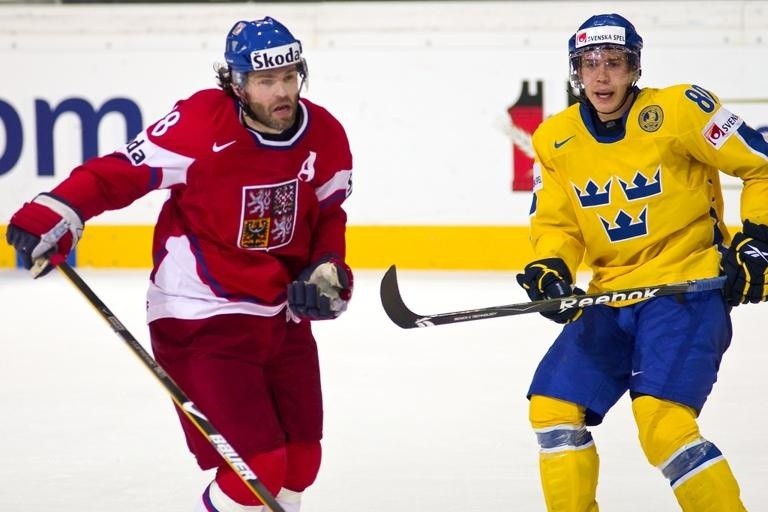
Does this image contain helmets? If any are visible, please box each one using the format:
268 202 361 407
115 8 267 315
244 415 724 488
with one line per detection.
223 15 309 88
567 13 644 72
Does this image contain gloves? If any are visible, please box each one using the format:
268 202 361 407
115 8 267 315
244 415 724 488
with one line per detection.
716 229 768 307
515 255 587 325
4 191 87 279
284 254 356 322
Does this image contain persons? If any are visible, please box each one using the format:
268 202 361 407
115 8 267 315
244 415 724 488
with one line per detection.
513 12 768 512
4 14 354 512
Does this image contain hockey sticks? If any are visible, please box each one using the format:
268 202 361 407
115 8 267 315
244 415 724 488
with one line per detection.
380 265 728 328
